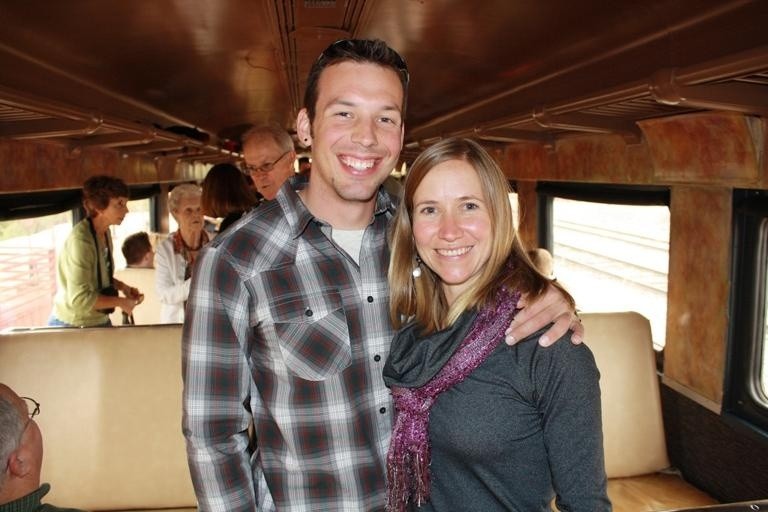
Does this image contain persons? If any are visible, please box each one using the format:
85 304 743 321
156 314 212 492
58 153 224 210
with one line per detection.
151 183 212 325
0 383 81 512
384 136 612 512
179 37 584 511
198 119 313 238
46 175 146 328
121 231 155 269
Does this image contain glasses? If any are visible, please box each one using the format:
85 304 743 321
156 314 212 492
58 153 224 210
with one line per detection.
243 150 291 172
4 396 40 474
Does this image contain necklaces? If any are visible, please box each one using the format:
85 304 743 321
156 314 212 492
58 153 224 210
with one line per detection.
179 229 203 251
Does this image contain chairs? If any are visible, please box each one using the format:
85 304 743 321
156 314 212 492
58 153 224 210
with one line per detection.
573 311 725 510
